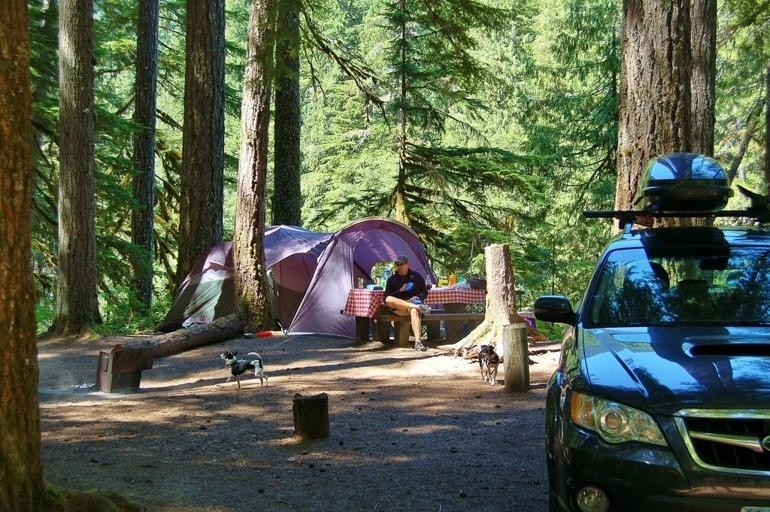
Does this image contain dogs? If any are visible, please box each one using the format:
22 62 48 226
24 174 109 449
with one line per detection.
478 344 499 386
220 350 269 390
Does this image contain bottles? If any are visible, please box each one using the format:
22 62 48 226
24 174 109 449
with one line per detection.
374 270 391 287
426 273 465 289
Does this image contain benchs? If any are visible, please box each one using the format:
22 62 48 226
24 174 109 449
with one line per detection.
341 307 370 337
383 311 537 345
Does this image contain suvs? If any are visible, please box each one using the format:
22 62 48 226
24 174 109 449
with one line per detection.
531 154 770 512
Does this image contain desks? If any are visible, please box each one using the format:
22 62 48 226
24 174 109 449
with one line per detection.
348 289 485 343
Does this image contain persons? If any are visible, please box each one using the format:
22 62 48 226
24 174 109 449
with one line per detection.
383 254 432 351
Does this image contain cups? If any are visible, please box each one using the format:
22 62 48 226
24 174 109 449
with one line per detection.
357 277 365 288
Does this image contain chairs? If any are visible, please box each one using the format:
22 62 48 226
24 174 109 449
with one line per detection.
603 262 680 324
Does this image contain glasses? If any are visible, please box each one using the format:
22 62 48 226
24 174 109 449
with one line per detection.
396 263 404 267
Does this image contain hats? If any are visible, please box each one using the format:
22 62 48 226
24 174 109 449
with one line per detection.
395 256 408 264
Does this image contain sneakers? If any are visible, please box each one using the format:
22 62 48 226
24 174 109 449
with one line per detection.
413 340 427 351
419 303 432 317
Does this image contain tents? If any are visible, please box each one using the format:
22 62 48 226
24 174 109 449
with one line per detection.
154 214 448 343
